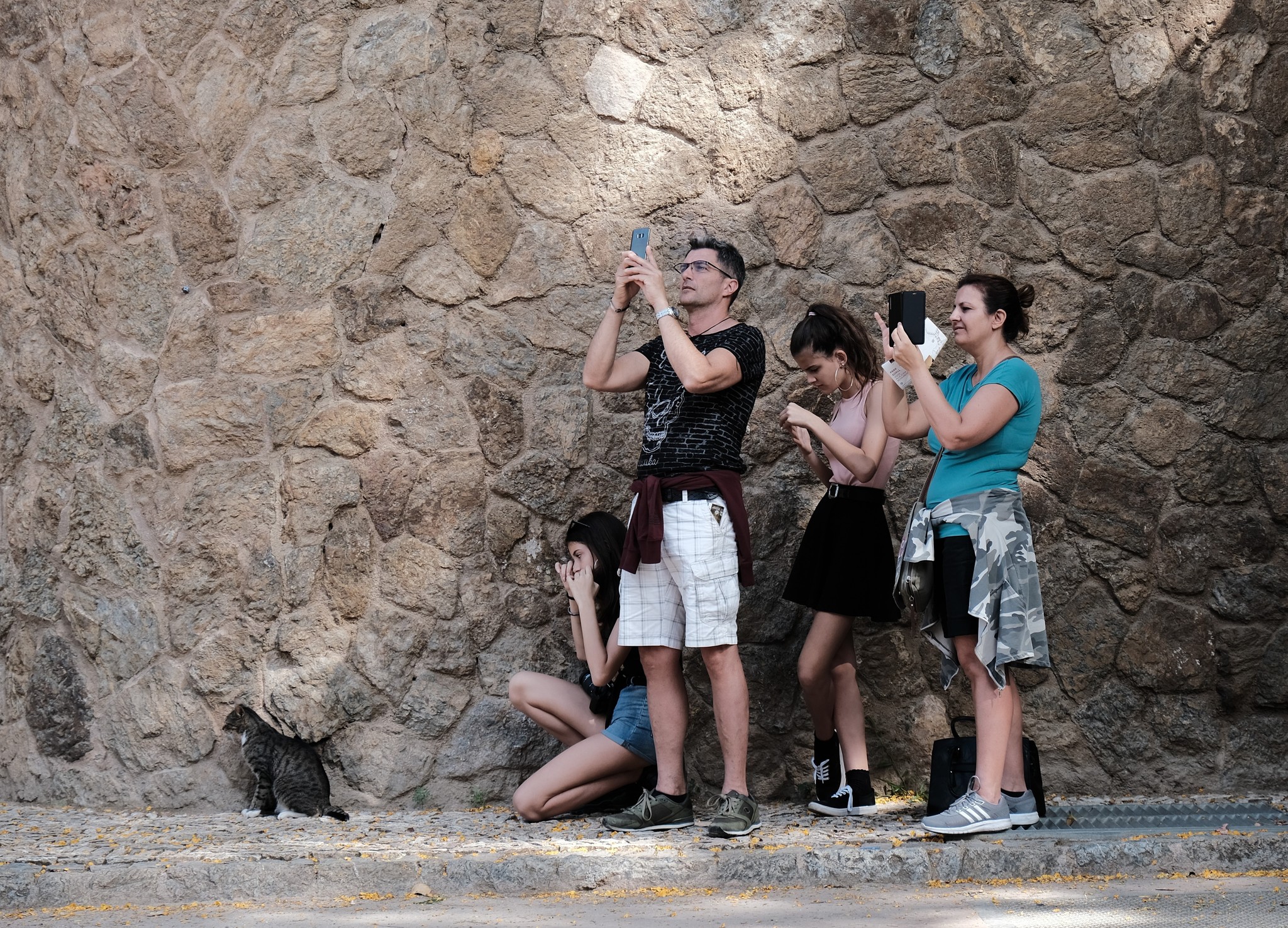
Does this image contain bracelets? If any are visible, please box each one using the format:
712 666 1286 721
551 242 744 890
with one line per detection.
568 606 580 616
566 592 575 600
609 297 631 313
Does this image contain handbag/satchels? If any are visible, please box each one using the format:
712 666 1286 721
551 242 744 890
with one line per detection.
925 716 1047 818
892 501 930 615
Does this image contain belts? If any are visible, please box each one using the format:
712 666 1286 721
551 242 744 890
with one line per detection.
662 491 706 503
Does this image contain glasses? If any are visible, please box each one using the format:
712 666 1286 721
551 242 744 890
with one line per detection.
673 260 732 279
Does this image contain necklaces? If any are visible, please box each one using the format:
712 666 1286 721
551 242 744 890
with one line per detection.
687 317 732 337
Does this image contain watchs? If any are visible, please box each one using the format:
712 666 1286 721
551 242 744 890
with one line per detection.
654 306 679 324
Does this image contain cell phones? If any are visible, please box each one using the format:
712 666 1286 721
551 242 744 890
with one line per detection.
630 228 651 267
889 290 903 346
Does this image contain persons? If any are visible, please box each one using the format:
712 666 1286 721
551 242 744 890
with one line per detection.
508 510 658 822
584 238 765 835
873 273 1051 833
779 303 900 816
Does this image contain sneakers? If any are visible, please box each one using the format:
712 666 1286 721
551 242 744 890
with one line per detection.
1001 789 1040 825
808 785 878 817
921 776 1013 834
707 790 762 837
811 728 846 801
601 787 695 832
